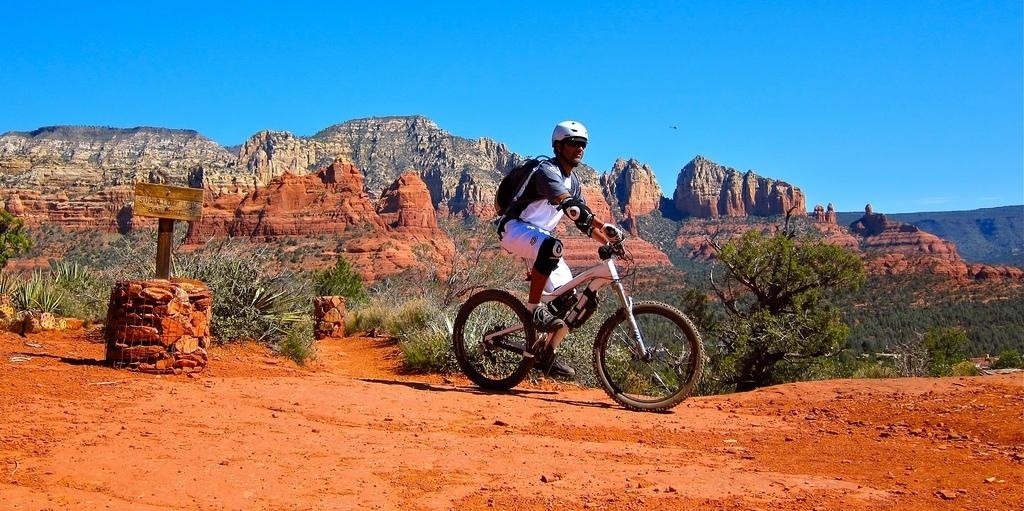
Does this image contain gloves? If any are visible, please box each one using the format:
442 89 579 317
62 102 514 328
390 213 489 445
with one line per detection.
600 223 622 242
604 241 627 260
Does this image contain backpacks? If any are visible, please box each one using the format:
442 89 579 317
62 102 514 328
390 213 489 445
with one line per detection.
494 154 567 216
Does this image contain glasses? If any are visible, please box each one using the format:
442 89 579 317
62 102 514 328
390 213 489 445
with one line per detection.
566 142 587 148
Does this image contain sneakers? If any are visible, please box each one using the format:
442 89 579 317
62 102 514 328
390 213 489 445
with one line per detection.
543 359 575 378
531 306 565 332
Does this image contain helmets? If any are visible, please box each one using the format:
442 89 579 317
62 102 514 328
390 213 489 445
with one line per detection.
551 120 588 147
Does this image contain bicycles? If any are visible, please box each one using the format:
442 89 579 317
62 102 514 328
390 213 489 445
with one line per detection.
453 226 705 412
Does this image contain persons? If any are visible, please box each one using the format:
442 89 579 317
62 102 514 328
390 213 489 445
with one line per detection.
494 118 628 377
